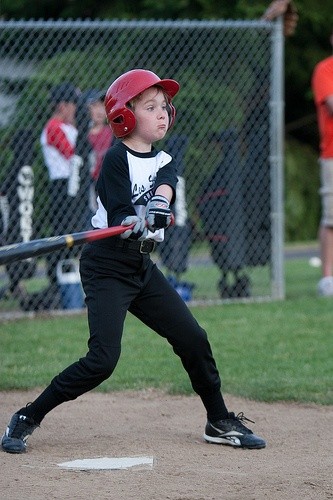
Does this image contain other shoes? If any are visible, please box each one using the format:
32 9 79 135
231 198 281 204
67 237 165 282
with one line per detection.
318 276 333 296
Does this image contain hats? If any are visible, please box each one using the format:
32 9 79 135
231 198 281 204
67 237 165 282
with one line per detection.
80 88 108 108
50 82 85 103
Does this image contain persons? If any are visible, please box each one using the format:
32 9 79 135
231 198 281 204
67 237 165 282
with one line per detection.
40 82 252 309
0 69 266 454
312 54 333 296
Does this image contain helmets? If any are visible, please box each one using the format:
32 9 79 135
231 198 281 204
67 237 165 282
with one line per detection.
103 68 181 139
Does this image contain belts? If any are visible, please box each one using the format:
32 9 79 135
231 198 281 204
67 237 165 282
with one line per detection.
90 229 158 254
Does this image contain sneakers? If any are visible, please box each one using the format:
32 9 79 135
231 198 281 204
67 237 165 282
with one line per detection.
1 402 39 453
202 412 266 449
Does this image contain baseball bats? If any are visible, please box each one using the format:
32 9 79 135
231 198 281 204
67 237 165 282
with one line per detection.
0 212 175 266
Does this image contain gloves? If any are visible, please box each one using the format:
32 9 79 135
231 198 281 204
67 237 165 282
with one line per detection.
121 215 147 242
146 196 171 232
